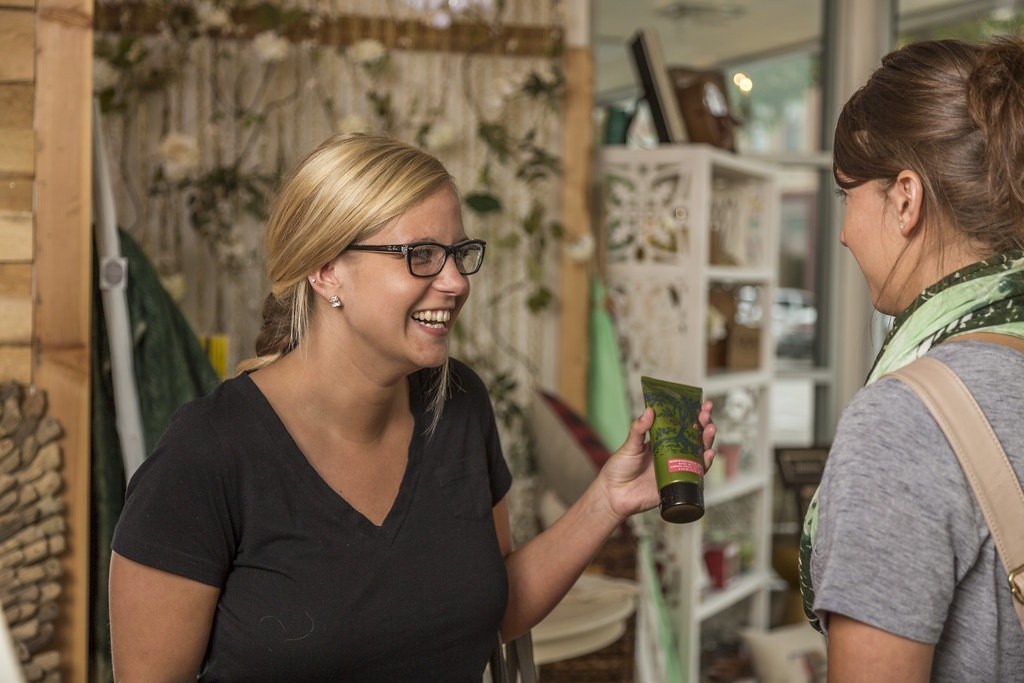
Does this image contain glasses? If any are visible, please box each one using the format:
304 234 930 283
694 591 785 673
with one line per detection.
343 238 486 278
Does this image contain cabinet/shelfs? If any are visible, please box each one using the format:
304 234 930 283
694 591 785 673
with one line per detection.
601 144 783 683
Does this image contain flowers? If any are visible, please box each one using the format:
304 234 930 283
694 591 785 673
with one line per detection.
101 1 594 447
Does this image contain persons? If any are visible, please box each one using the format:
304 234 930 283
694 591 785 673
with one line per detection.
797 37 1024 683
108 132 716 683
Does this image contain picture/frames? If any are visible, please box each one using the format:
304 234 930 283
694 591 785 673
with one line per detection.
624 25 688 145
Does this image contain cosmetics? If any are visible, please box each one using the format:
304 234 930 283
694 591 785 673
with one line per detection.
640 375 706 524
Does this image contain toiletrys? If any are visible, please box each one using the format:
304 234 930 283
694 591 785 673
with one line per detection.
640 375 706 525
